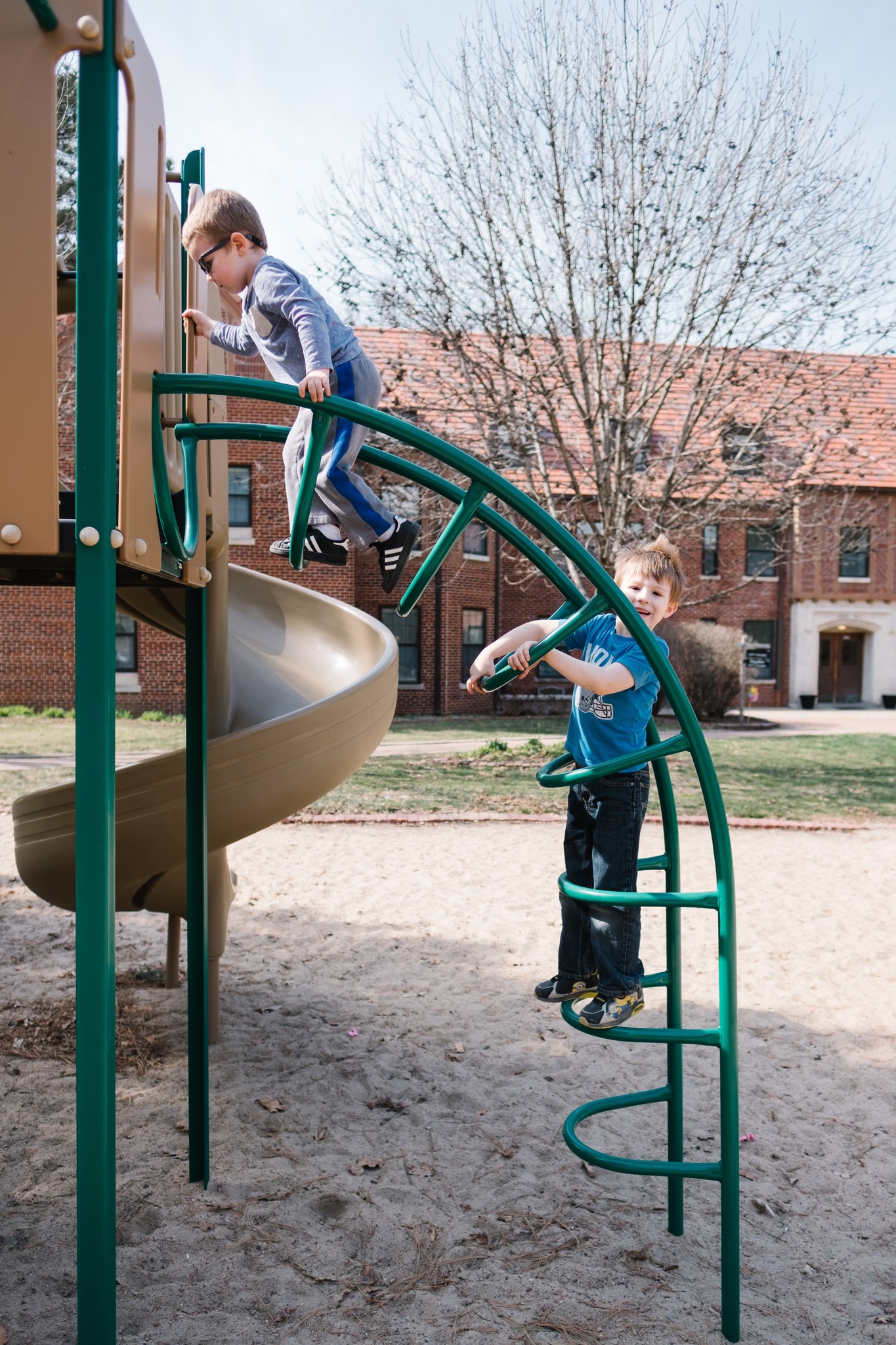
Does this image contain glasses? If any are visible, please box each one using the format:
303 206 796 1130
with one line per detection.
198 235 247 274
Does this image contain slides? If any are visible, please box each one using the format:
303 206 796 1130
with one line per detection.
12 563 399 960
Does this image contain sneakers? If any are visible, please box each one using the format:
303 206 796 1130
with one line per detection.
268 526 350 566
369 514 421 595
533 967 600 1003
578 976 646 1030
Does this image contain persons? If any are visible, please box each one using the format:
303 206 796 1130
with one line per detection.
180 187 421 593
466 534 683 1028
